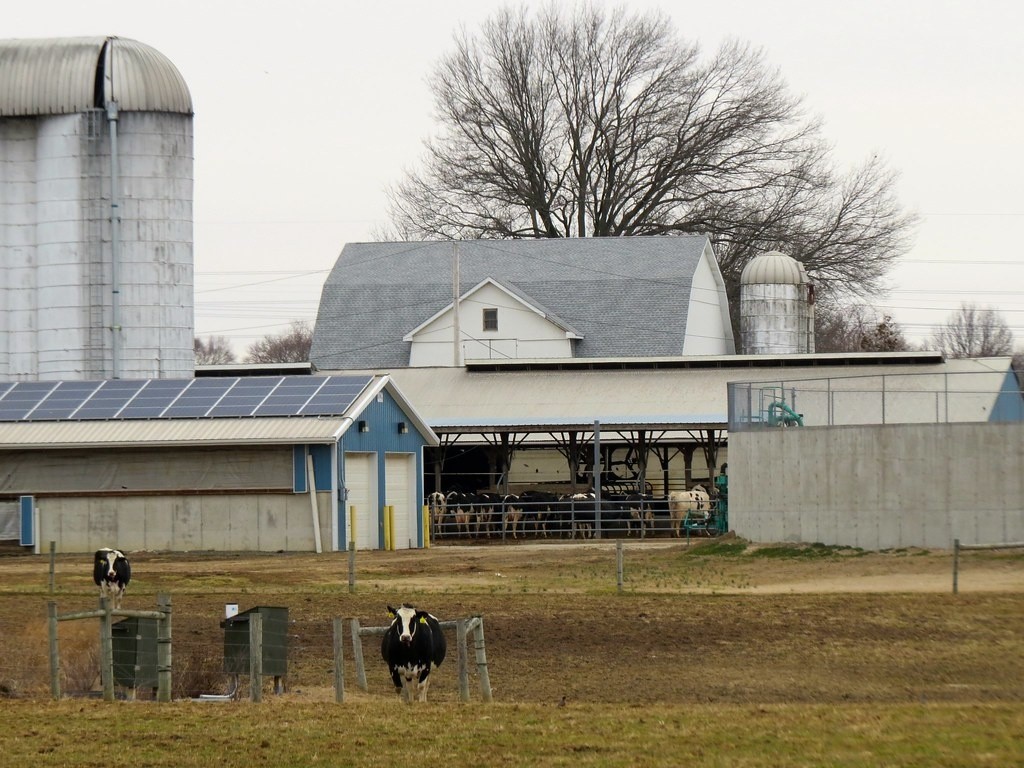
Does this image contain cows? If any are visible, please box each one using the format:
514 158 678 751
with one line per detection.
94 547 131 610
426 482 714 539
381 603 447 703
